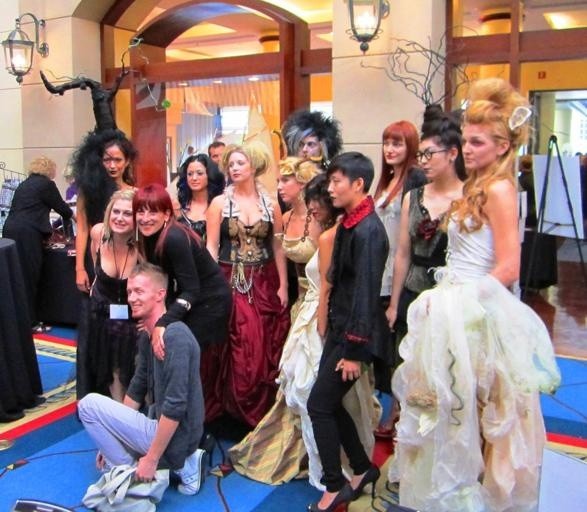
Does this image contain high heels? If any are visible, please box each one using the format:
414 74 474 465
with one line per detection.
351 463 380 501
202 433 214 467
304 483 352 512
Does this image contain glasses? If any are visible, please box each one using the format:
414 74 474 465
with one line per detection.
413 148 448 163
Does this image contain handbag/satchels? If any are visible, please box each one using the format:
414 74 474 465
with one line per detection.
82 469 170 512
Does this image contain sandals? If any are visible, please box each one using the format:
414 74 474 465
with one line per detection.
374 418 396 437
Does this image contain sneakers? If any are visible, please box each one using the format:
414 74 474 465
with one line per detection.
177 447 207 495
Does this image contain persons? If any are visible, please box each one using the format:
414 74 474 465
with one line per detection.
388 77 520 511
519 151 587 292
3 156 75 332
77 263 208 498
72 102 464 512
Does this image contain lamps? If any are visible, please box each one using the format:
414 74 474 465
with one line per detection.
345 0 390 56
1 11 50 84
